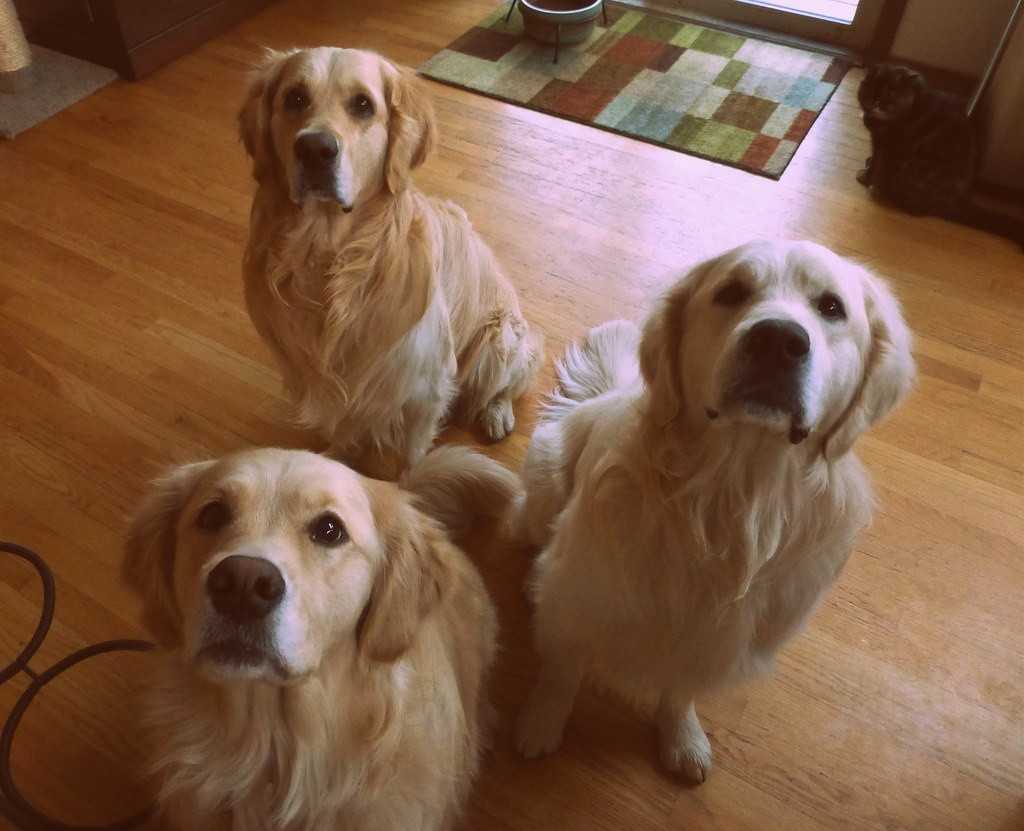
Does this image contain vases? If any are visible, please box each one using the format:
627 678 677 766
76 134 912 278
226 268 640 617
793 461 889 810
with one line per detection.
516 0 602 46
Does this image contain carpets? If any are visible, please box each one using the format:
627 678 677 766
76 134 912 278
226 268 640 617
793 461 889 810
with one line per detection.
0 45 120 138
418 0 854 184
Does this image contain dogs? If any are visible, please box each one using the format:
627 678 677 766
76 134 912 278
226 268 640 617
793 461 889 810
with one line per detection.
119 443 531 827
519 236 920 786
235 45 542 480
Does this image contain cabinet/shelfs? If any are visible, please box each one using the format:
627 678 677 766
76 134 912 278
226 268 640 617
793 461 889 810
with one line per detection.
13 0 273 83
